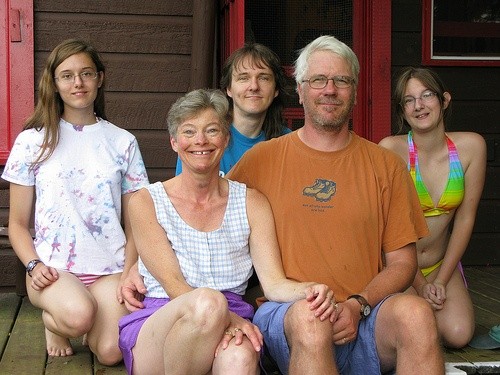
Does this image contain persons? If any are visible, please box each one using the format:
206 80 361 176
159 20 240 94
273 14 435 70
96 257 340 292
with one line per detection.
372 68 487 351
120 33 447 375
171 42 294 192
0 36 154 366
120 86 339 374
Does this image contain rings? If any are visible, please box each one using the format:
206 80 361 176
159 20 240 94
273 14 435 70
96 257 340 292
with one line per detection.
224 329 233 337
234 328 242 335
334 303 339 311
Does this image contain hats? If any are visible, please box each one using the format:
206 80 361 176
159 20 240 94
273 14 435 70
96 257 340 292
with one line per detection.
469 324 500 350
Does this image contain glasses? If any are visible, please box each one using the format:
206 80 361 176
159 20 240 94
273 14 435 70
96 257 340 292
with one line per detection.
398 92 437 107
53 71 99 80
301 74 355 89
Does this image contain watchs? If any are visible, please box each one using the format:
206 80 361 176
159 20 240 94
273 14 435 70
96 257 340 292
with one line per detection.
346 294 372 321
26 260 44 278
